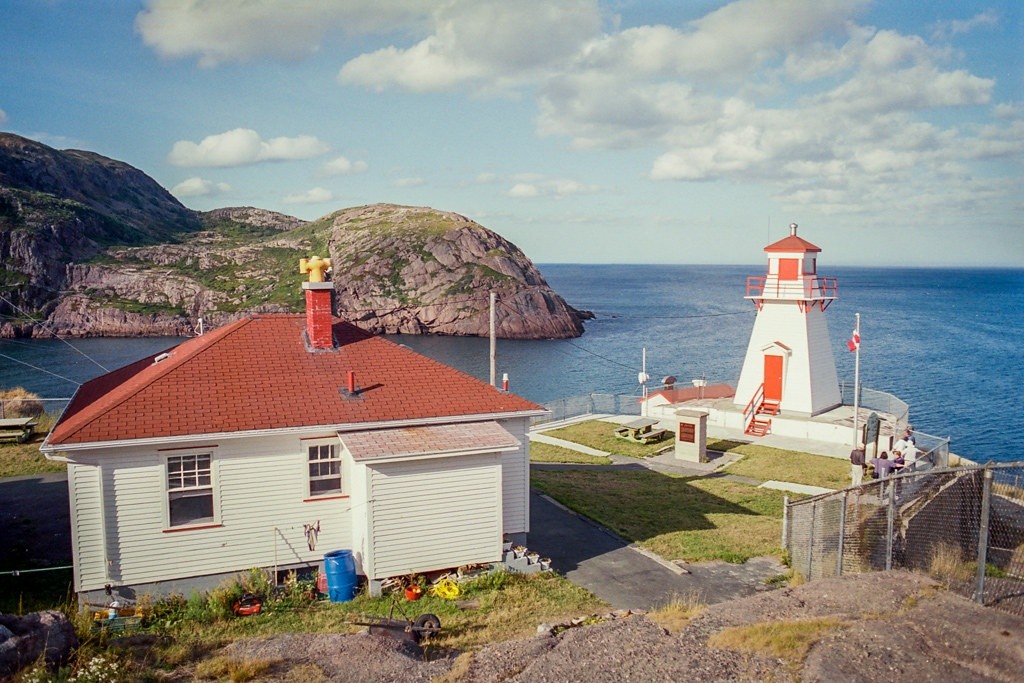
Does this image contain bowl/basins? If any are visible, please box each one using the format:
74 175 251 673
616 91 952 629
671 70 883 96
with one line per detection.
406 586 422 600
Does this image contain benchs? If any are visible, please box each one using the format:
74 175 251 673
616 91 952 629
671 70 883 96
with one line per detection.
0 422 39 446
614 427 627 438
639 429 667 444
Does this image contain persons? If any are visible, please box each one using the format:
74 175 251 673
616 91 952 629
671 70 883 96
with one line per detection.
870 429 927 499
850 443 867 491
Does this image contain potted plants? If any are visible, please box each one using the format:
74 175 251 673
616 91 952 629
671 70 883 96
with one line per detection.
503 539 552 569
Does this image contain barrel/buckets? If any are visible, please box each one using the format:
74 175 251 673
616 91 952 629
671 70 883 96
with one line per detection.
323 550 358 602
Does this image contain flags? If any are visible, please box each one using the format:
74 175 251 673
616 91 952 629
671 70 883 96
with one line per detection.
848 312 861 351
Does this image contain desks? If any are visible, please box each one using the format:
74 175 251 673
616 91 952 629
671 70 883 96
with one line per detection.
622 417 660 443
0 417 33 442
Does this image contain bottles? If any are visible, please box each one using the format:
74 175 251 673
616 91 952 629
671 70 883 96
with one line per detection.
107 599 121 620
